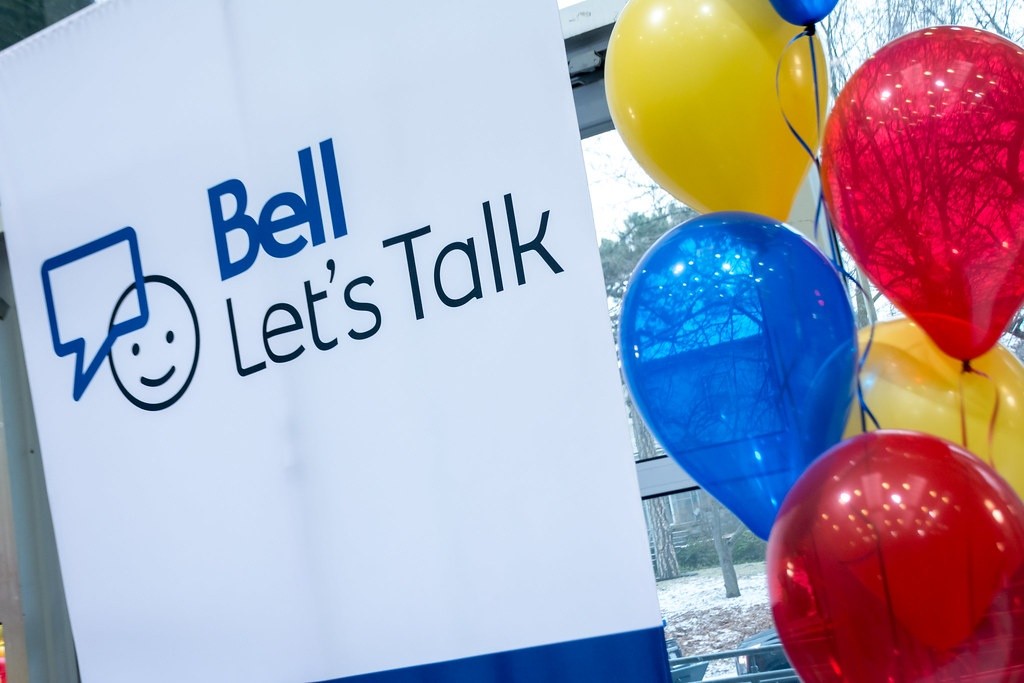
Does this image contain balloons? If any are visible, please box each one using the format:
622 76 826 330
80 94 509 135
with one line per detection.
603 0 1024 543
765 429 1024 683
